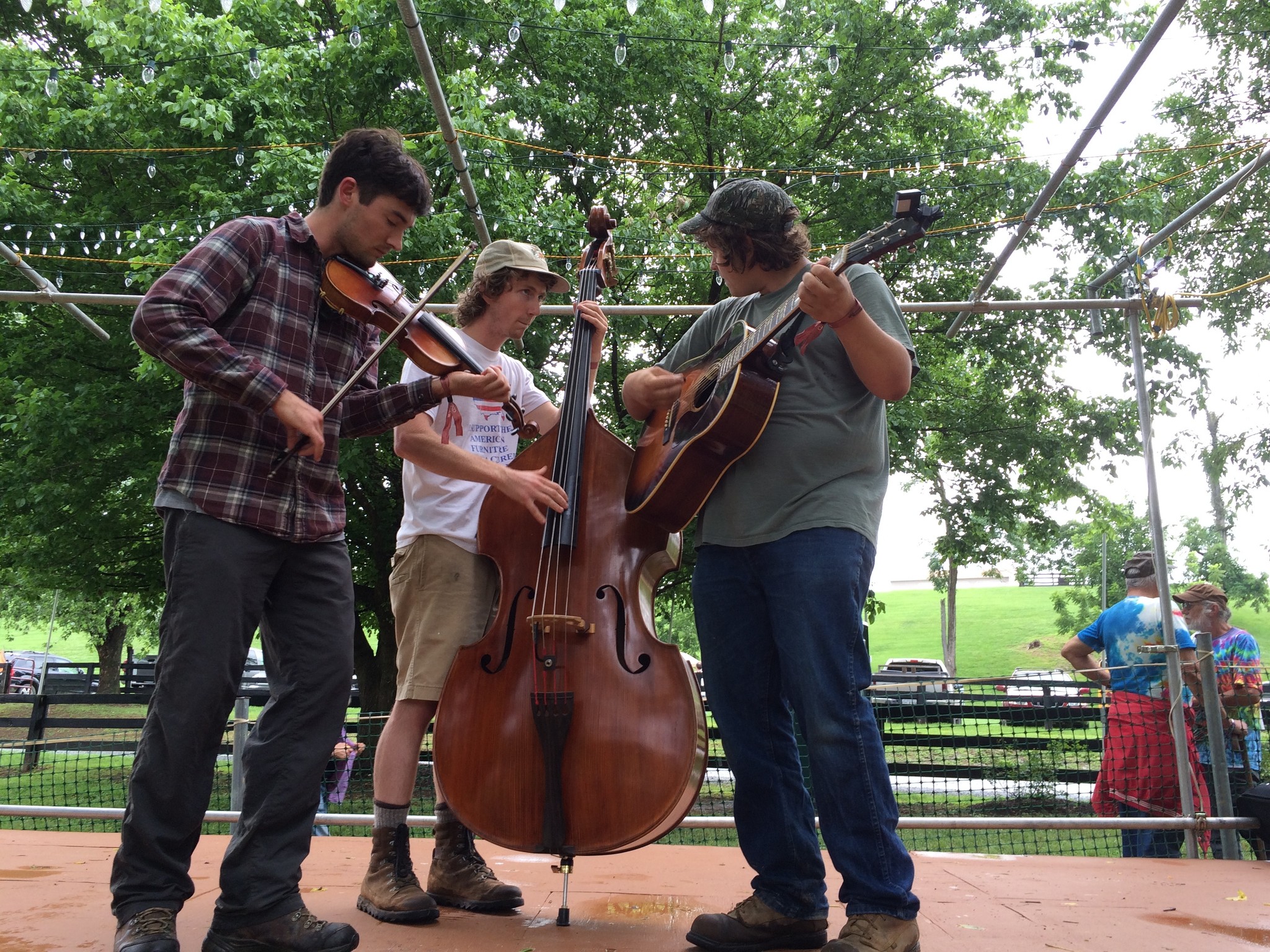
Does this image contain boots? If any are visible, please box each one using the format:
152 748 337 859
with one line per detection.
357 823 440 923
425 820 525 913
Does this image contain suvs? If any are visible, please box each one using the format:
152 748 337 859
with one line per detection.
2 651 100 695
122 658 156 687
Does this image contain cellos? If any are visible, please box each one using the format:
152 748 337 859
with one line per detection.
431 198 711 929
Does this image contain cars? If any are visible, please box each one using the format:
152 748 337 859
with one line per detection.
995 668 1091 730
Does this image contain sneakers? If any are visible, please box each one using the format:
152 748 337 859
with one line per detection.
202 907 359 951
821 913 922 952
685 895 829 951
113 909 180 952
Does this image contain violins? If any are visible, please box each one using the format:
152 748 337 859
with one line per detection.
318 248 545 438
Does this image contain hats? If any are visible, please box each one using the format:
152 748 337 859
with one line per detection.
1124 551 1177 579
473 239 571 294
1172 584 1228 607
678 176 798 235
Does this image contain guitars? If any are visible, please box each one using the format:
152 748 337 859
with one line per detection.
619 186 942 533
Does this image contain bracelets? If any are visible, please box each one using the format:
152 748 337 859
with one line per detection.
1107 681 1111 689
794 296 863 356
590 361 600 369
311 736 365 836
1230 718 1235 729
440 374 463 445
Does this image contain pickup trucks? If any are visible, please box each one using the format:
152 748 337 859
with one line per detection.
870 658 964 723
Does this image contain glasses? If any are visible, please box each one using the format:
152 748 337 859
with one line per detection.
1182 601 1217 611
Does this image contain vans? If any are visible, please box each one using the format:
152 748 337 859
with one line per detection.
241 647 270 688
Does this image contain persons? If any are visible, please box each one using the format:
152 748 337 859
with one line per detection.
1171 584 1258 860
1062 550 1247 860
109 129 509 952
619 178 919 952
355 239 607 924
